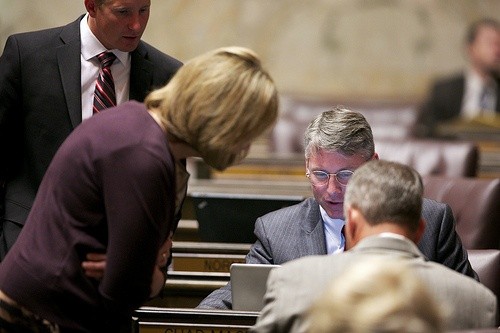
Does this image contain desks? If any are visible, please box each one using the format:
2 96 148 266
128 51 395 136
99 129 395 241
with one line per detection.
185 135 315 244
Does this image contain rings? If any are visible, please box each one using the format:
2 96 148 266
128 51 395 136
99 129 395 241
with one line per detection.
162 251 168 258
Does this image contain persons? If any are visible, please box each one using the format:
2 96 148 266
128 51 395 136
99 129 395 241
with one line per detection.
0 45 279 333
250 159 500 332
405 16 500 142
194 107 480 312
0 0 185 264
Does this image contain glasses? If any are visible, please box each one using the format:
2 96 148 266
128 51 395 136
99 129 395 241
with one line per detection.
306 170 354 186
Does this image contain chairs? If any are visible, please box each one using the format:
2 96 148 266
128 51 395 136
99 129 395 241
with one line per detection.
268 98 500 328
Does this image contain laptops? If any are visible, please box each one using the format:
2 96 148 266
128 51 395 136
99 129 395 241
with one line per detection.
230 264 280 312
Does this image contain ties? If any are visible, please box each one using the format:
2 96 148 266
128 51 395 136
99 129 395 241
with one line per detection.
93 51 117 116
